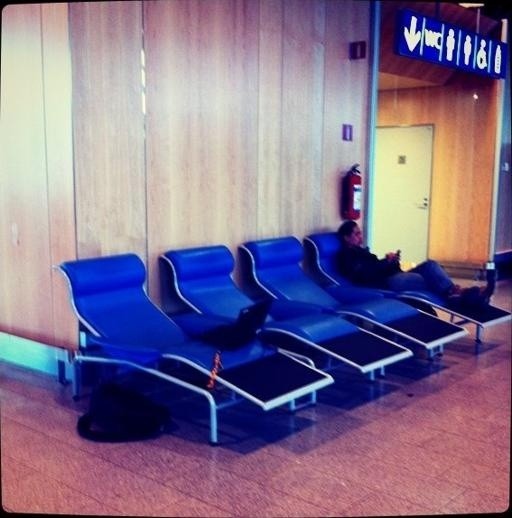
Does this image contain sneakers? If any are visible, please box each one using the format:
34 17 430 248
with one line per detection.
447 284 490 307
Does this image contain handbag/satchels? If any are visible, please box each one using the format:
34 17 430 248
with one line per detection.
77 381 170 442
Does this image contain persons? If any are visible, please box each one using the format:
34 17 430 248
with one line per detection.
338 219 495 310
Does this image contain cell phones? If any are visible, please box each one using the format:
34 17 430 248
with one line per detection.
395 249 400 256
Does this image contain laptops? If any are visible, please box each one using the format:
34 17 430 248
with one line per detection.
192 298 272 351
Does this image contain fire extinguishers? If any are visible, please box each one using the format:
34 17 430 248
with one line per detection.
343 163 362 220
